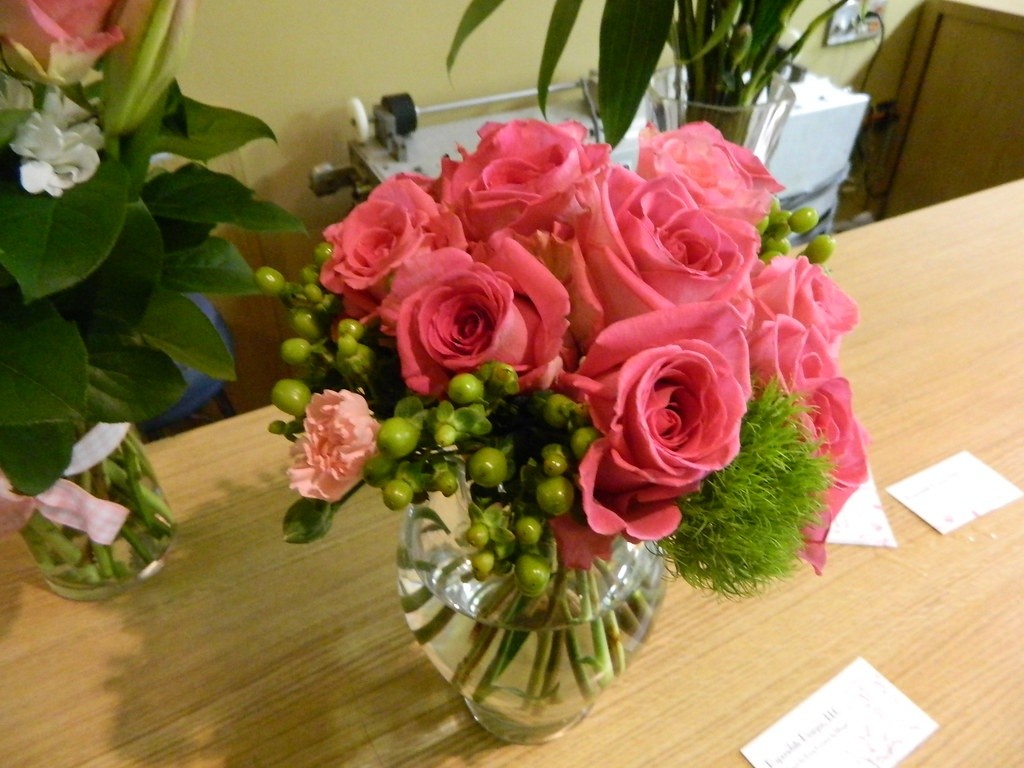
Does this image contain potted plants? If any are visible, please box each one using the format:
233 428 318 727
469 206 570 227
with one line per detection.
446 0 870 169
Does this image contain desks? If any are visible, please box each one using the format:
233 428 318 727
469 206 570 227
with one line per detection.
0 176 1024 768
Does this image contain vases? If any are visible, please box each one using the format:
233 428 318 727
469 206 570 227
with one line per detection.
1 421 177 601
397 461 667 745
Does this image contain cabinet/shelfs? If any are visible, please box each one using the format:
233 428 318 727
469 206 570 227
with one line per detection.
857 0 1024 221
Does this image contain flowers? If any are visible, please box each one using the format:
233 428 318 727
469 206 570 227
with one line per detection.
259 119 872 718
0 0 307 578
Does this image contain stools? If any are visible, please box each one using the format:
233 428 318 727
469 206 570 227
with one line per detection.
140 292 237 442
